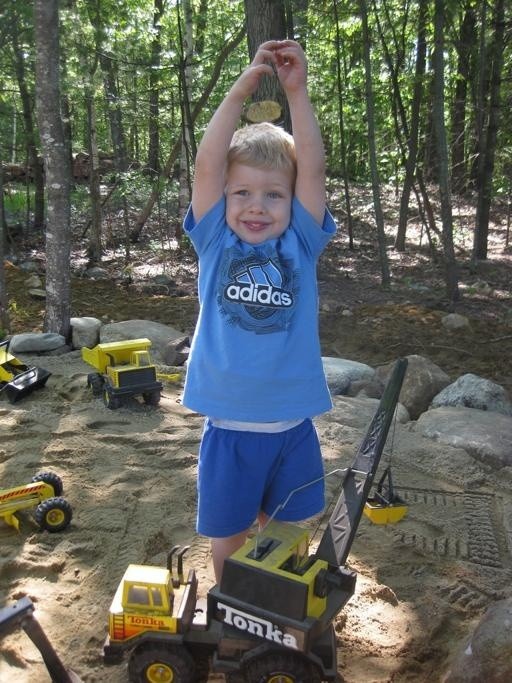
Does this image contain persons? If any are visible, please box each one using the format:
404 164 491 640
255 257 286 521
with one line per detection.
180 39 340 586
